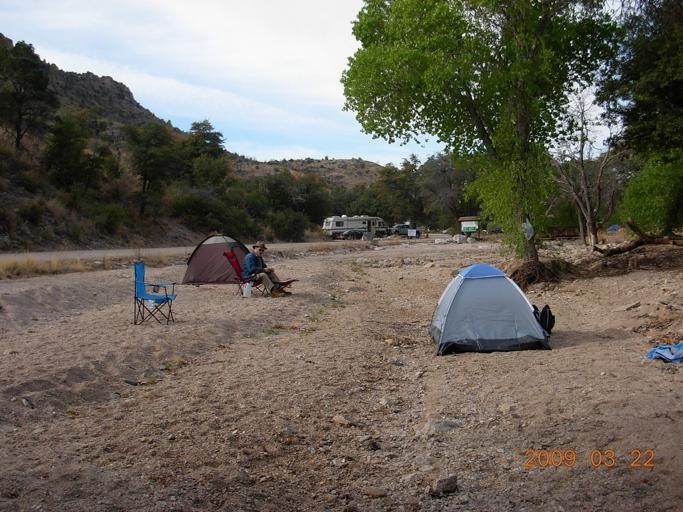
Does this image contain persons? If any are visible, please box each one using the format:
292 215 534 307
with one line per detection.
240 241 291 298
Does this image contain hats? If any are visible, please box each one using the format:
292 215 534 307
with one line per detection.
252 241 267 250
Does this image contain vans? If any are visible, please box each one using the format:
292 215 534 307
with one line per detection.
322 215 388 235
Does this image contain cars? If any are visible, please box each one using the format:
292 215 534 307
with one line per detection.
340 230 363 240
389 224 413 235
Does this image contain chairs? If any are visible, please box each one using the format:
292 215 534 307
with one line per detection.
223 250 299 297
134 261 177 325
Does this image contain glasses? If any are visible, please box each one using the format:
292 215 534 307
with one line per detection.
259 249 264 252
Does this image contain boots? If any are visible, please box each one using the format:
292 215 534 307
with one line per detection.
271 288 292 297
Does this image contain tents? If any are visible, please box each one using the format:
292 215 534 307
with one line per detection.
428 262 552 355
181 231 250 285
